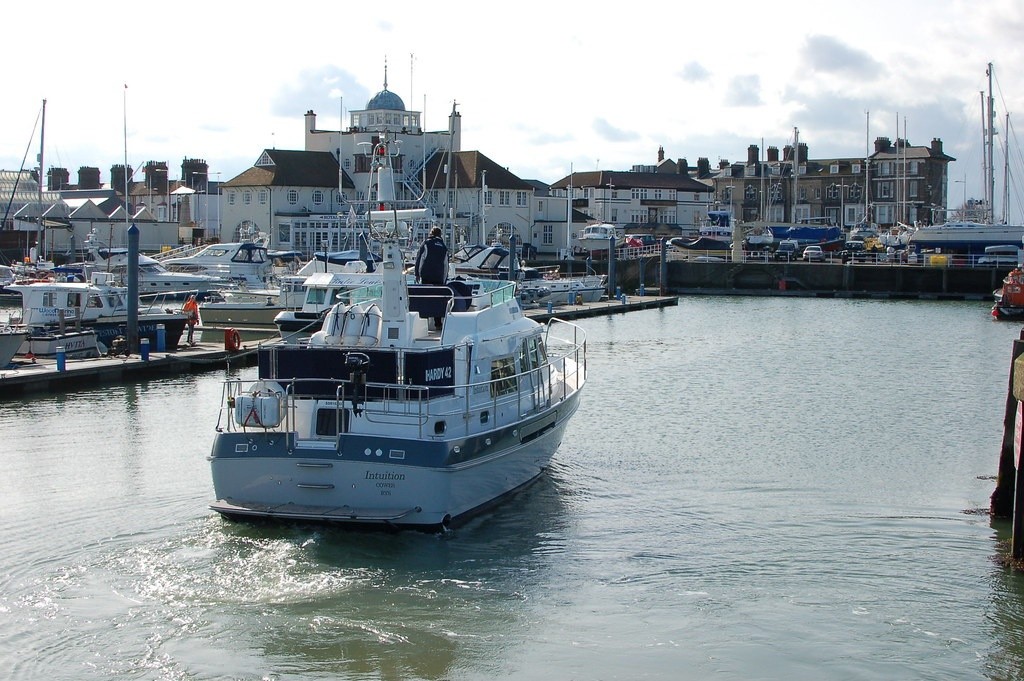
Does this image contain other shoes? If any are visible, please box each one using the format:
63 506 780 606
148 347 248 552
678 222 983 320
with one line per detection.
434 323 443 330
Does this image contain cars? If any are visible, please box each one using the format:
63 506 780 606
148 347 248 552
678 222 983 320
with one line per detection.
803 246 825 262
774 243 797 262
841 241 867 264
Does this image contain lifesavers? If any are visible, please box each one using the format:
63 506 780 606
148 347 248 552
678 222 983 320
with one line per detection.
245 381 288 425
227 329 241 351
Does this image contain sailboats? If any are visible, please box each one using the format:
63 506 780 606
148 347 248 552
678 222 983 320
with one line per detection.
670 63 1024 263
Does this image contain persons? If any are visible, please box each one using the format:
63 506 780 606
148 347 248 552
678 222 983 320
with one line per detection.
871 244 878 262
181 295 199 347
887 243 895 266
414 227 449 330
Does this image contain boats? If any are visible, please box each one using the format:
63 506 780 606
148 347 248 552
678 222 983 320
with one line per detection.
0 307 100 359
0 223 199 352
578 223 624 251
0 98 608 331
0 308 33 370
992 269 1024 321
203 94 589 534
